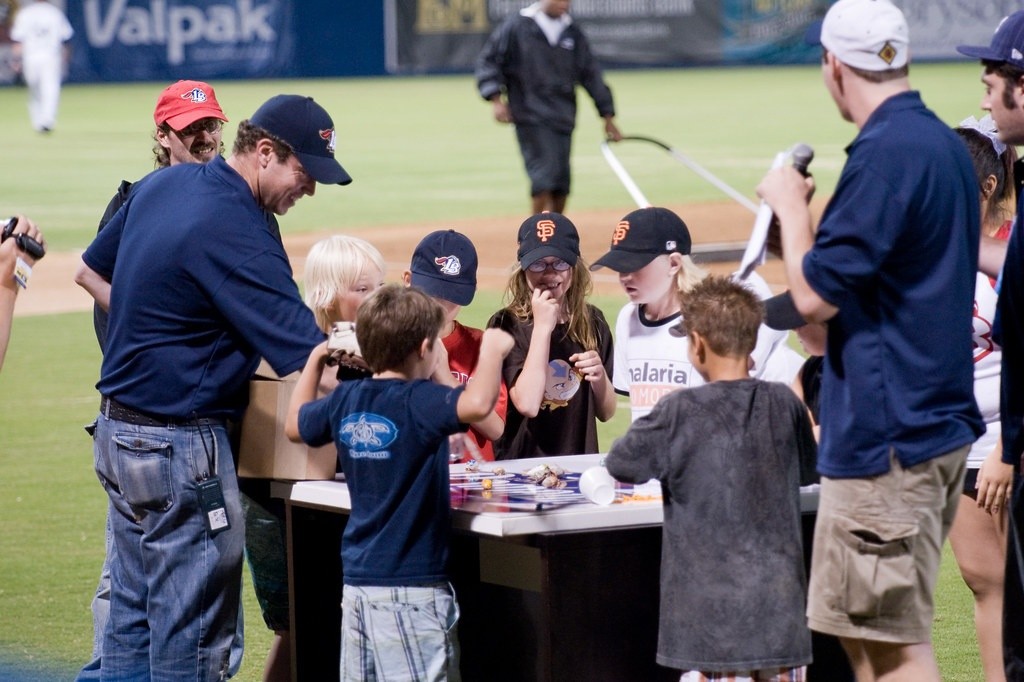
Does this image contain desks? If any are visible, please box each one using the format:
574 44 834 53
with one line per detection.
269 452 858 682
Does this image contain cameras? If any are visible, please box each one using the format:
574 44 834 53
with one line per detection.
2 218 18 243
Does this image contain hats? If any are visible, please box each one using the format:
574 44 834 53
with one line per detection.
956 10 1024 69
410 229 478 306
820 0 909 71
517 210 580 271
153 80 229 131
248 95 352 187
589 207 692 273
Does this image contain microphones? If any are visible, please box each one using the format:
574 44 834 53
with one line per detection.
763 145 813 252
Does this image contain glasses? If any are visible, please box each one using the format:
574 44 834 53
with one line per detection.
527 258 572 273
179 119 224 136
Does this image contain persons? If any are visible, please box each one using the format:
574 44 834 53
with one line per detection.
754 0 982 682
955 10 1024 681
0 213 45 371
473 1 620 215
242 201 822 682
82 75 229 681
74 96 342 681
936 118 1018 682
9 0 75 133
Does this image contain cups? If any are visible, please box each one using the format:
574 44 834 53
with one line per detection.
578 466 616 504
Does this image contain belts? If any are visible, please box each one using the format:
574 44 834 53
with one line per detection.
100 393 230 428
